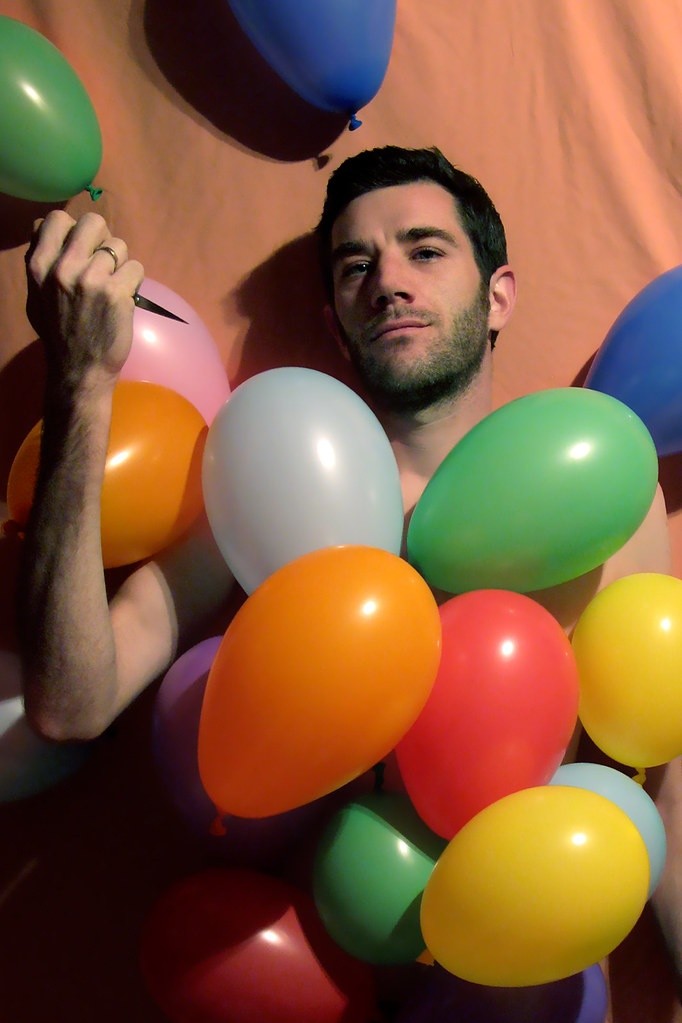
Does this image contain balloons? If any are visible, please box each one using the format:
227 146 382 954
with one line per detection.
140 546 682 1020
0 15 102 204
118 278 231 425
201 367 405 597
582 267 681 456
407 387 658 596
226 2 396 131
9 379 208 566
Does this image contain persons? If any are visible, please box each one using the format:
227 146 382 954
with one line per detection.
23 145 682 1020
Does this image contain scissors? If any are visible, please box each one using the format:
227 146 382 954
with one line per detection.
133 289 190 324
